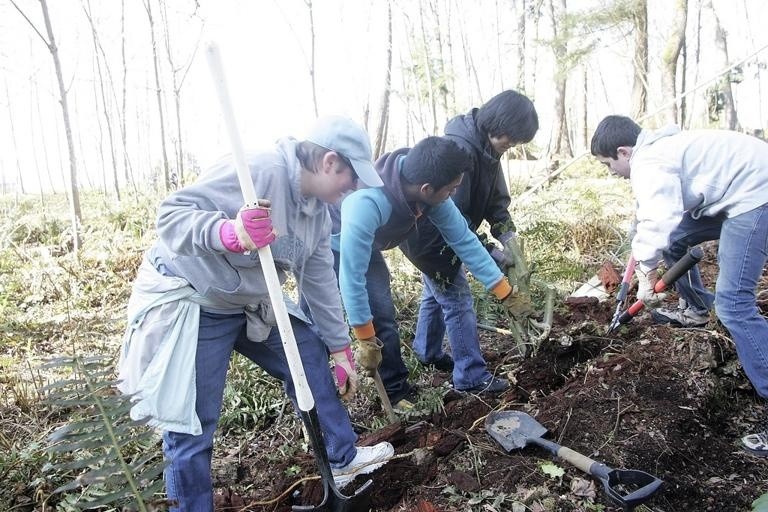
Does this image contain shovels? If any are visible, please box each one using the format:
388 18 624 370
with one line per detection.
485 410 665 506
206 45 372 512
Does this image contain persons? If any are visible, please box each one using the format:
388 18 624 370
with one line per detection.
398 88 543 399
589 113 767 455
117 120 394 512
295 135 540 460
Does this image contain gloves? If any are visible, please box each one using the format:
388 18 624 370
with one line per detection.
211 198 277 256
330 349 358 402
358 338 384 370
477 219 504 251
634 265 666 303
499 289 535 323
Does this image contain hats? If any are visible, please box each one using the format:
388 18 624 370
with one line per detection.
302 116 385 187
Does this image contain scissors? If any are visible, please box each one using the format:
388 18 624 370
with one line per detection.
602 243 703 340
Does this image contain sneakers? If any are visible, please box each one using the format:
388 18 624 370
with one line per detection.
650 297 711 330
330 441 395 490
453 371 509 392
380 397 417 415
420 351 454 373
739 429 768 456
